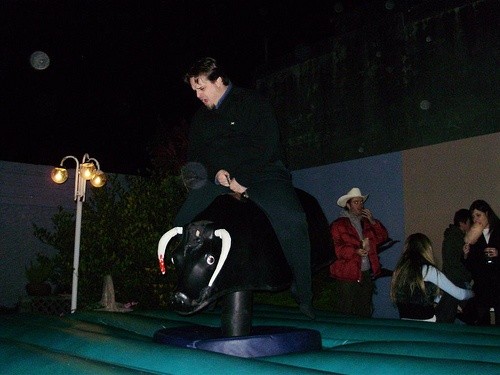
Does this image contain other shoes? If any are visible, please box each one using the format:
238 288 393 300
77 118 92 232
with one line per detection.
292 283 316 319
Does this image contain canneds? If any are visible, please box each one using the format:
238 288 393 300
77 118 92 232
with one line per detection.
484 248 493 264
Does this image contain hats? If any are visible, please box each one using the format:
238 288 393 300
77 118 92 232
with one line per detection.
337 188 369 207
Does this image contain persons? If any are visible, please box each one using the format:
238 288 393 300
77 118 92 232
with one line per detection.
433 208 474 326
329 187 389 315
174 58 310 319
462 199 499 326
390 233 473 322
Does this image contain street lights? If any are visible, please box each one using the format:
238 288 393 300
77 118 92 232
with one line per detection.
50 152 107 313
